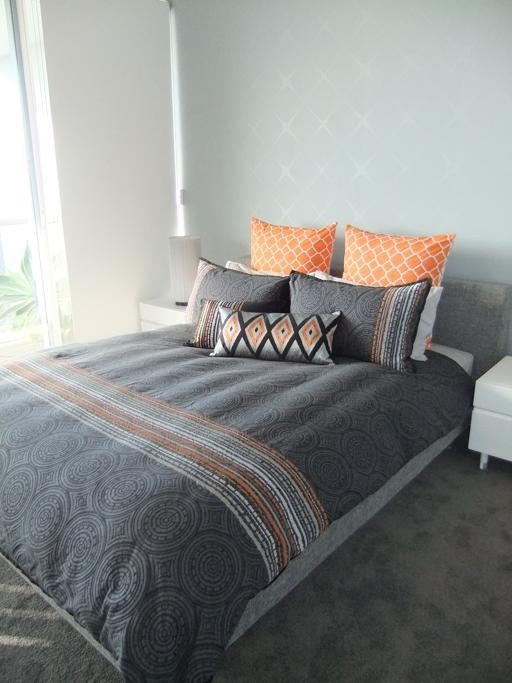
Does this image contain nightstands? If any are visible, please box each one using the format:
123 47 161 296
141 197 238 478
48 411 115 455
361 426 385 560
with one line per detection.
138 301 190 331
465 353 511 472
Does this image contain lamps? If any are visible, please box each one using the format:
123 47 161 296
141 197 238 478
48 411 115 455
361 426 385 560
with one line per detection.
167 234 203 306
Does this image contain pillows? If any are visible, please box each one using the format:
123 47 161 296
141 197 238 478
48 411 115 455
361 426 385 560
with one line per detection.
248 215 339 278
186 258 291 327
224 260 290 279
314 270 444 364
339 223 458 287
180 299 289 349
287 269 434 373
208 304 342 367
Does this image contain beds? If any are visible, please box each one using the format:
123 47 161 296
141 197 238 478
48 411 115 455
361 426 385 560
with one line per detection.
1 268 511 683
